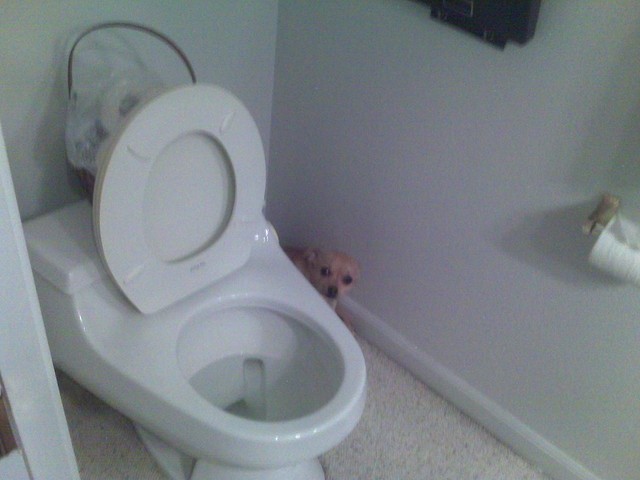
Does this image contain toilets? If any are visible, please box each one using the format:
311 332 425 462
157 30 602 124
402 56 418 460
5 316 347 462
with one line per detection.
20 83 370 479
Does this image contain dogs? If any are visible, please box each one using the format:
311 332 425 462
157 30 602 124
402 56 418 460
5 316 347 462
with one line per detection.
283 246 360 335
